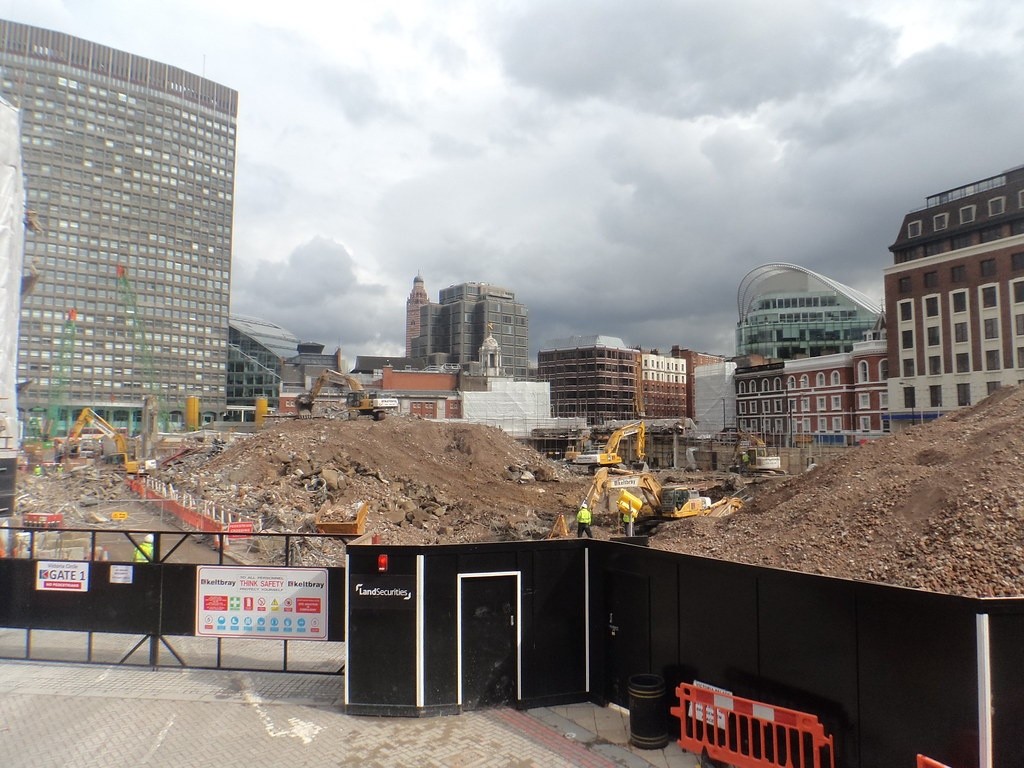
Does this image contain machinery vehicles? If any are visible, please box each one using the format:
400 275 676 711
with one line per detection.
726 429 791 476
563 418 650 476
54 408 142 477
580 468 712 532
295 367 401 422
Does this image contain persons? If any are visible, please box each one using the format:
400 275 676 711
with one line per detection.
623 514 635 537
741 452 750 468
576 504 593 538
34 464 41 474
56 464 64 473
45 464 50 471
131 534 153 562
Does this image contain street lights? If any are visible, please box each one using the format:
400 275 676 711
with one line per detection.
899 381 916 427
722 398 726 433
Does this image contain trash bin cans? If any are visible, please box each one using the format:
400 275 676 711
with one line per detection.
627 673 669 750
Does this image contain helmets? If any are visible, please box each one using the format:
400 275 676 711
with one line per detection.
582 504 587 508
145 534 154 543
